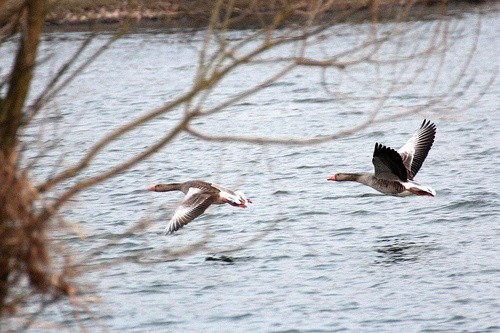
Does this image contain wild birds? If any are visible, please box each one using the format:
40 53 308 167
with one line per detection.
327 118 437 197
147 180 253 236
